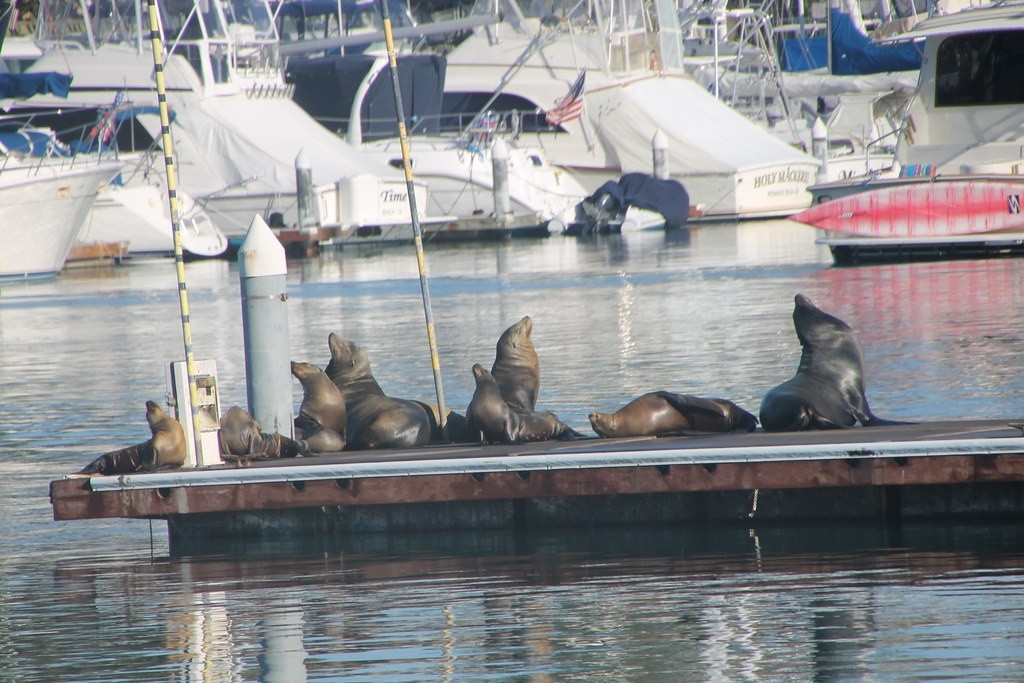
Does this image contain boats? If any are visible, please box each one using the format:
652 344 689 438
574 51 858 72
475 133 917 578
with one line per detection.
0 0 1024 293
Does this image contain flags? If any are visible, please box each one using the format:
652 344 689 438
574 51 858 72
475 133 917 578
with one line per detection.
544 67 586 125
